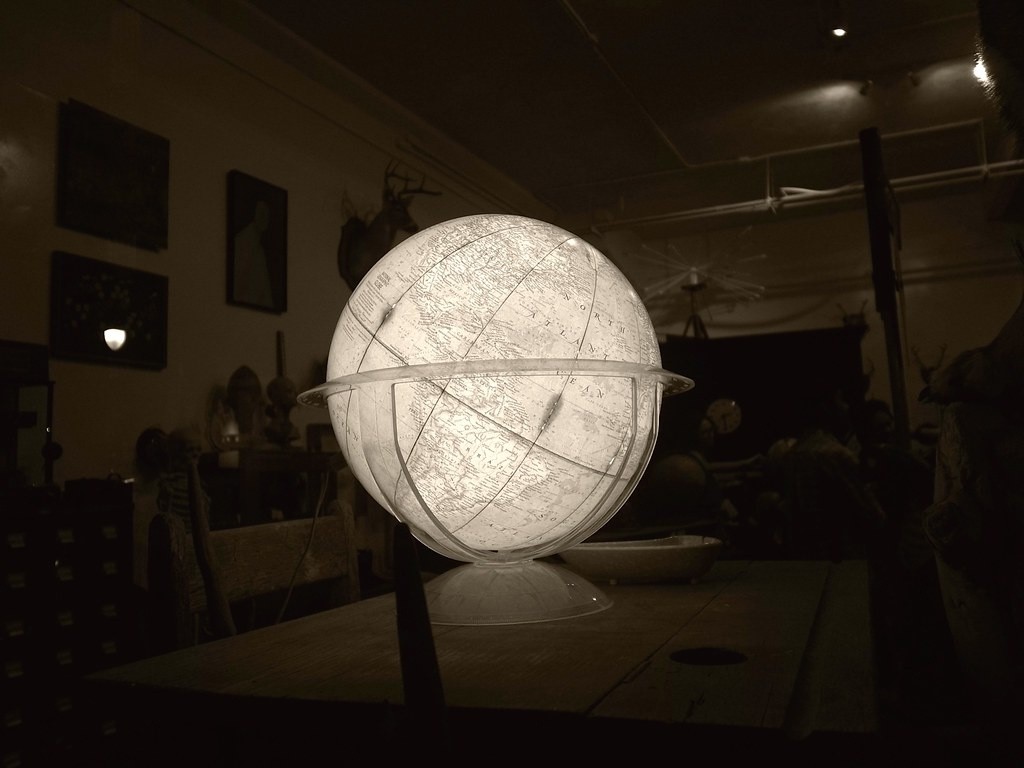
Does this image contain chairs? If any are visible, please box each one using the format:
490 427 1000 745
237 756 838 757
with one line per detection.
187 459 362 638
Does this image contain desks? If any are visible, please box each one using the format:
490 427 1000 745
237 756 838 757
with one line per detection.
80 557 868 768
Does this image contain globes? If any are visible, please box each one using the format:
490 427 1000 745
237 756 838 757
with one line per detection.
295 210 697 631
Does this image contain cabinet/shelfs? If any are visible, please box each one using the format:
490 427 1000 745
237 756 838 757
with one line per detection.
0 339 141 768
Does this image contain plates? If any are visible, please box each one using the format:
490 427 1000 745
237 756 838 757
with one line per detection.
555 534 724 581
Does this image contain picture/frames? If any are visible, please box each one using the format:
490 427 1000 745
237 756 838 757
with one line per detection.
53 251 171 372
226 169 289 313
62 97 170 252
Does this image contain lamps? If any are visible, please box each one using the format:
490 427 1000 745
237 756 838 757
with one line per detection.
294 216 696 625
909 72 923 87
860 79 874 97
